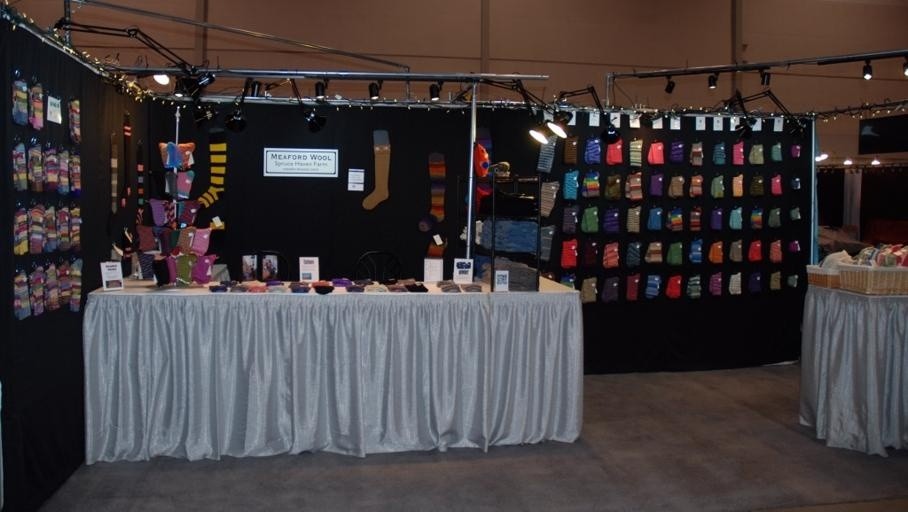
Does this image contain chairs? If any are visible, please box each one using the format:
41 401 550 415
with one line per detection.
355 251 401 284
247 250 289 285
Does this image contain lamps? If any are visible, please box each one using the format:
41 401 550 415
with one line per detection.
663 69 772 97
45 16 217 101
481 76 617 149
861 58 875 81
244 72 446 129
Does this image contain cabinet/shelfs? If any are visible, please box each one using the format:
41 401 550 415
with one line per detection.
457 174 541 292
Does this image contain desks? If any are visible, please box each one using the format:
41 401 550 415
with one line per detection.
179 358 184 361
805 284 908 458
85 265 583 462
